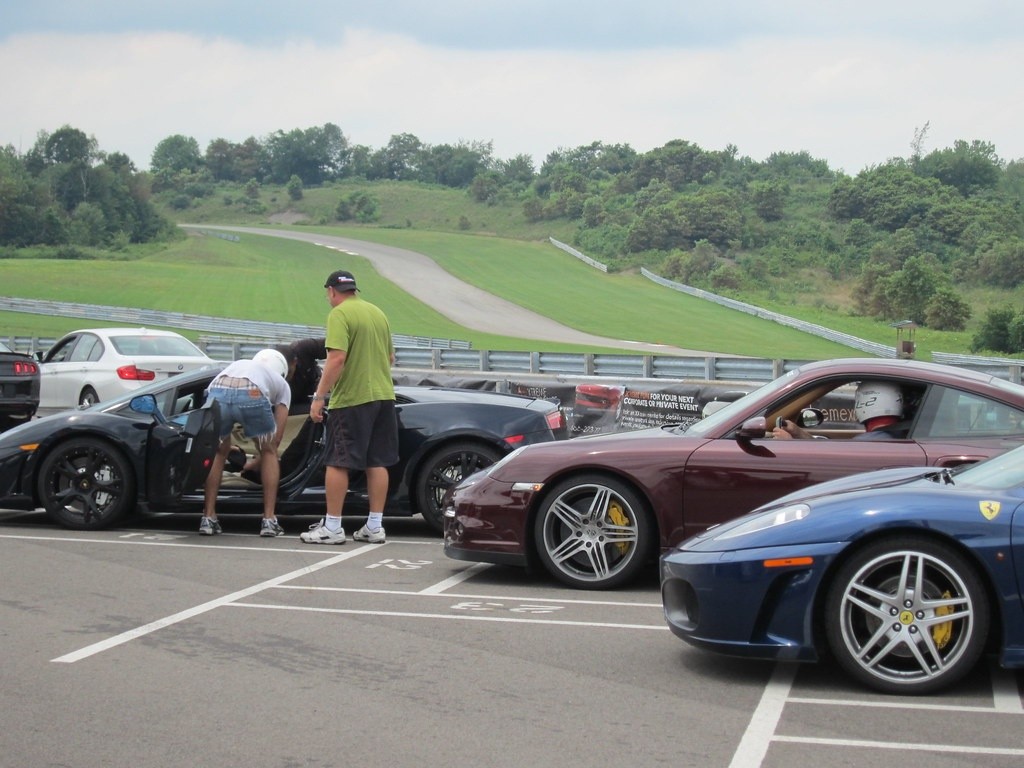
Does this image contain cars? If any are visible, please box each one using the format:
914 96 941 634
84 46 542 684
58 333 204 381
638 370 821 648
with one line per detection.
30 327 238 427
0 340 42 432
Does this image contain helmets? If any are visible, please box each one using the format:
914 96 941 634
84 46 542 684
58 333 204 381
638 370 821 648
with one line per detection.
856 379 903 423
252 348 289 381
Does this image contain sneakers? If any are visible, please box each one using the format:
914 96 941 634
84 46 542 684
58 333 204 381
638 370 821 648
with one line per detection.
260 515 285 536
352 523 386 544
198 517 223 535
300 518 346 544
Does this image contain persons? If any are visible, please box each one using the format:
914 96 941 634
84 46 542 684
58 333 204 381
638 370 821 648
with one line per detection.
771 381 908 439
271 338 326 416
199 349 291 536
300 270 399 545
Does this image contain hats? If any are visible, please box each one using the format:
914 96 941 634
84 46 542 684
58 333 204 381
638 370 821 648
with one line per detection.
324 270 361 292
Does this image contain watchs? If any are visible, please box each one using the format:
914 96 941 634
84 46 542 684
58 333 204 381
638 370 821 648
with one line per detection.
312 392 325 400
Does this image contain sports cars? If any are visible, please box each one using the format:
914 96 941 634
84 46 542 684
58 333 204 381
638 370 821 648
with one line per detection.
440 358 1024 590
658 438 1024 695
0 364 568 540
